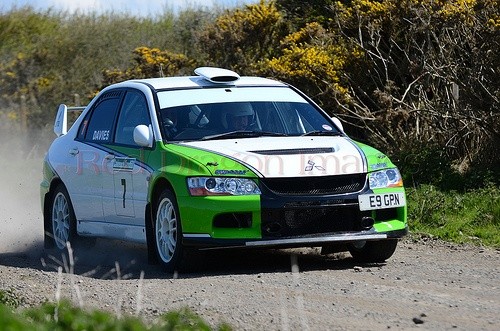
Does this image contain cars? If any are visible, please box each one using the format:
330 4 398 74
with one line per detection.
38 65 410 273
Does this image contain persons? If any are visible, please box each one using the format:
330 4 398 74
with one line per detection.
221 102 255 133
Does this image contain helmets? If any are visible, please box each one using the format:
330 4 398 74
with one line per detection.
221 103 256 128
